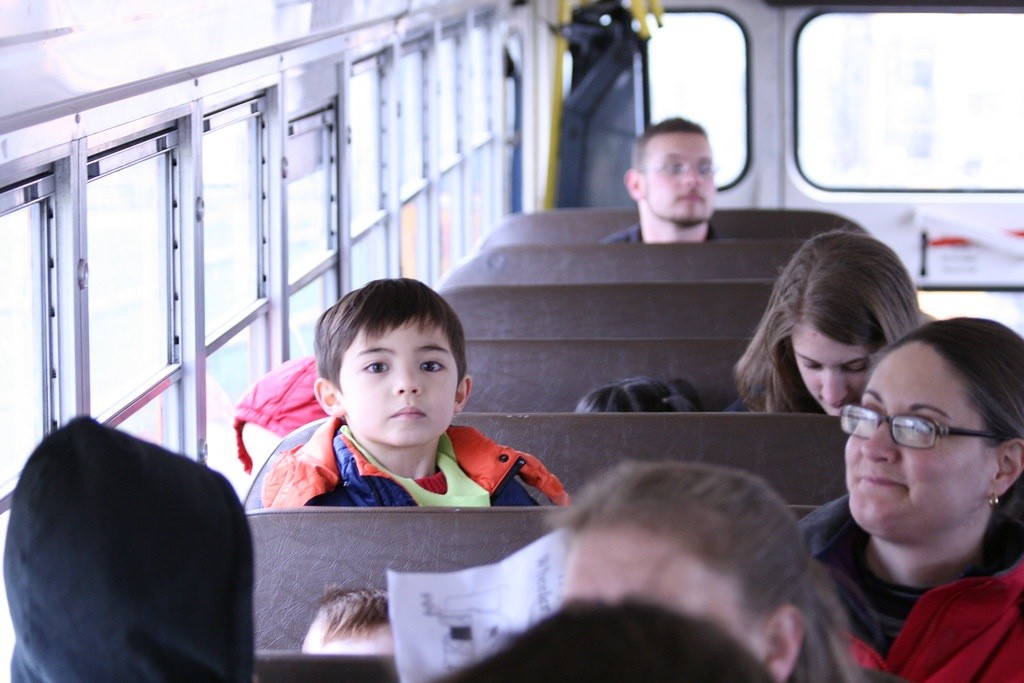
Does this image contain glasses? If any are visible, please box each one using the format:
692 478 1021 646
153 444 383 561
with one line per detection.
840 404 994 449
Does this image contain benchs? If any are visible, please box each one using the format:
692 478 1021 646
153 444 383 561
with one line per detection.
243 207 875 683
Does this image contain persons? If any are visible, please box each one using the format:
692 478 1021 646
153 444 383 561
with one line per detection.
260 231 1024 683
600 117 717 241
4 418 256 683
